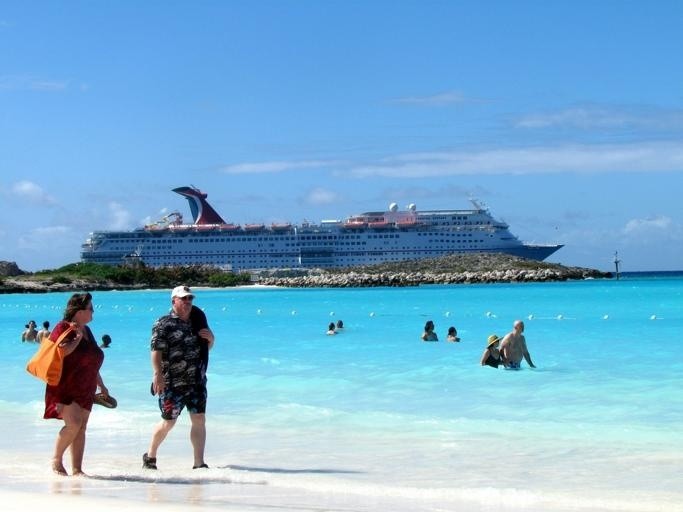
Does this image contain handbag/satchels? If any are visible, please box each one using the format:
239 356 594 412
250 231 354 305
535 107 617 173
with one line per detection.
24 320 77 388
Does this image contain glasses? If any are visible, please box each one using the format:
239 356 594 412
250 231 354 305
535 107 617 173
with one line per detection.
179 295 195 301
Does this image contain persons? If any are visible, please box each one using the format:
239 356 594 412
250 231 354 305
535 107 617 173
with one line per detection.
100 335 112 348
480 321 536 370
446 327 461 343
421 321 439 341
326 320 343 334
21 321 39 344
141 286 215 469
37 321 50 344
44 292 109 477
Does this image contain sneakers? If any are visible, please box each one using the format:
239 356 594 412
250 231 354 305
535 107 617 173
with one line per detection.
92 391 117 409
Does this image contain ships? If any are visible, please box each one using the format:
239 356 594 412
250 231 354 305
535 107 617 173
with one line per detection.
79 186 564 269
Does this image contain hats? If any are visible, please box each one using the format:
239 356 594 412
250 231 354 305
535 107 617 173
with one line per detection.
170 285 197 300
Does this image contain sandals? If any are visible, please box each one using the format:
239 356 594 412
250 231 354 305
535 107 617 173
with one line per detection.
142 452 158 471
